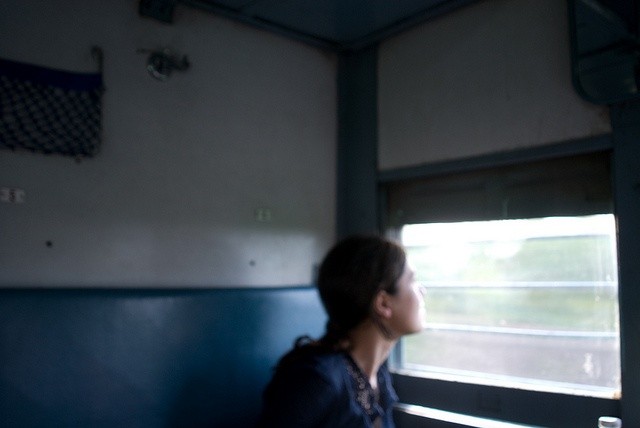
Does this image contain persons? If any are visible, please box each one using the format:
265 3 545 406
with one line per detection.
271 236 425 427
